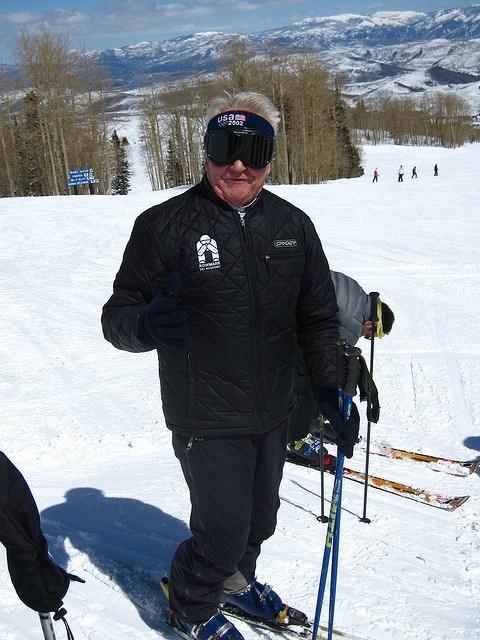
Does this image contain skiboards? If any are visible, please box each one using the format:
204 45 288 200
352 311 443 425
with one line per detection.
285 424 479 510
83 547 368 640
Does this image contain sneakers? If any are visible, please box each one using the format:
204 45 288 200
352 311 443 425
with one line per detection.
167 607 244 640
286 432 328 466
218 579 282 619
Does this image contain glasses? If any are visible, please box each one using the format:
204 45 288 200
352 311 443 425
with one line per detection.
206 129 273 169
364 336 371 340
364 336 371 339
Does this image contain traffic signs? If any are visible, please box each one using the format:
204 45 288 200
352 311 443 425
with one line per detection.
66 168 99 185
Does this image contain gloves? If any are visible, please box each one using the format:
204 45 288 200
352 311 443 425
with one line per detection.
312 384 360 458
137 269 192 352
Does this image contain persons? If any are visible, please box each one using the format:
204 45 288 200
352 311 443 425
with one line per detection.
100 88 361 639
411 167 417 178
434 164 439 176
286 270 395 471
398 165 405 182
372 167 379 182
